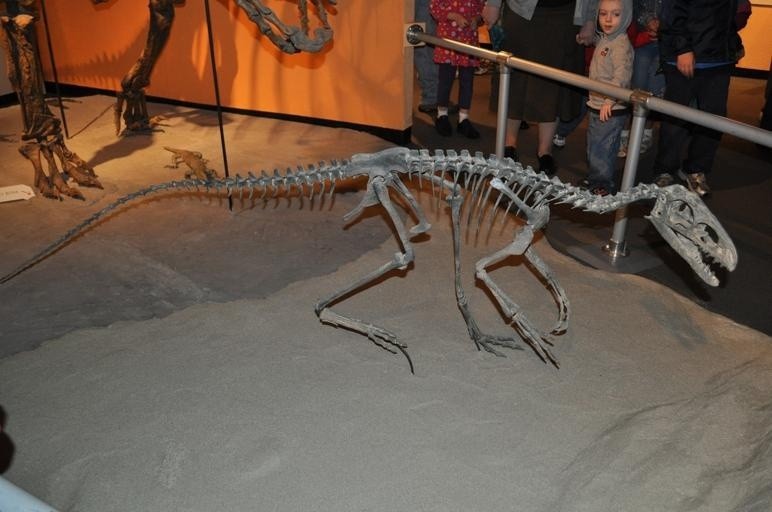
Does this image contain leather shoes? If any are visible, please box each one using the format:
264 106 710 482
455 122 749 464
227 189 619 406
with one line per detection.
437 115 478 138
538 153 555 176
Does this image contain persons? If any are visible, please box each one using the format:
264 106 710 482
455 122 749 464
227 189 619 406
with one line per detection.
413 0 752 197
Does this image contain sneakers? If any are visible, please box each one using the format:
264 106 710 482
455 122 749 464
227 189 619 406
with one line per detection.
655 168 710 197
